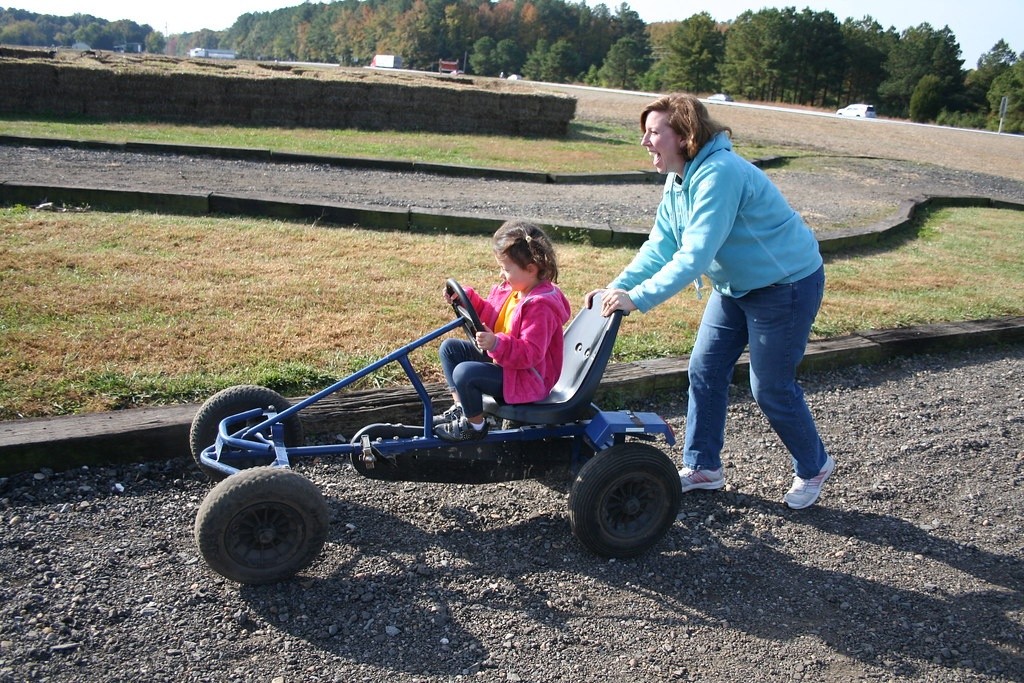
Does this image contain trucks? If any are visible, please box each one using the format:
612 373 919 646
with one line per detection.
371 54 403 69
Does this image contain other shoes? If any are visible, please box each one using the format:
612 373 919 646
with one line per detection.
434 416 490 443
432 405 463 426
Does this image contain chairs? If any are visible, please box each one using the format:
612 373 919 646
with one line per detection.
481 292 626 425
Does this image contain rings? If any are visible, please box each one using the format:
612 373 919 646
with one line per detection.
607 302 611 307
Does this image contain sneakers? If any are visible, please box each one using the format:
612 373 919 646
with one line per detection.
783 453 835 510
678 463 726 493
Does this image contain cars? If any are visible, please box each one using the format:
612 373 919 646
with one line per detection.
836 104 877 119
707 94 734 102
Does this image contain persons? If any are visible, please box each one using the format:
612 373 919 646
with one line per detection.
433 219 571 443
585 93 834 509
